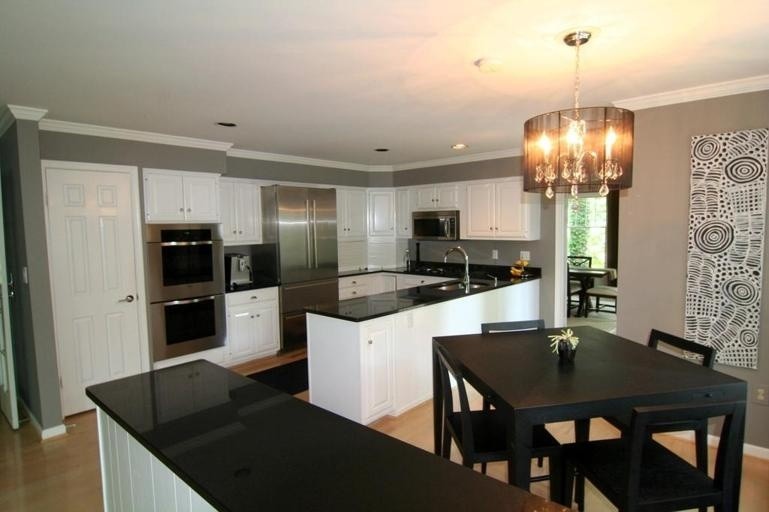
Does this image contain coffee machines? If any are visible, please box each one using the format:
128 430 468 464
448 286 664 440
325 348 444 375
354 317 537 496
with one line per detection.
225 250 255 287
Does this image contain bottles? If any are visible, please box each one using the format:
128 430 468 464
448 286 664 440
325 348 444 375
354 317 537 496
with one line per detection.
403 250 411 270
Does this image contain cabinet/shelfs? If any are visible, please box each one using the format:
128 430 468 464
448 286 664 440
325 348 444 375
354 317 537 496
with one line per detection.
337 177 542 241
338 275 455 302
218 182 261 246
229 284 281 361
142 173 218 224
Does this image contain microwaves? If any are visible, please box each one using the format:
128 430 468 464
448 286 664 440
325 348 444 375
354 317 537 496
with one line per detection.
412 209 460 241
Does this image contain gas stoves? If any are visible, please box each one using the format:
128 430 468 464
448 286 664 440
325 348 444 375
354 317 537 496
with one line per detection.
412 264 447 275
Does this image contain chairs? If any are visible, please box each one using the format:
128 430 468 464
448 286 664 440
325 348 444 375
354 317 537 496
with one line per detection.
430 335 558 502
478 321 594 503
586 328 718 511
558 395 747 512
567 256 617 318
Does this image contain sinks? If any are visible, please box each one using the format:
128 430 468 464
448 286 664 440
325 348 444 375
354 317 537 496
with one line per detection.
434 281 491 291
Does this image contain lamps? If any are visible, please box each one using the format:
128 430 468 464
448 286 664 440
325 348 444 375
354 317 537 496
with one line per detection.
524 32 634 199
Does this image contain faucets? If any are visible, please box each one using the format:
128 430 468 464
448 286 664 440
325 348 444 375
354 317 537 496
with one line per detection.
442 245 470 277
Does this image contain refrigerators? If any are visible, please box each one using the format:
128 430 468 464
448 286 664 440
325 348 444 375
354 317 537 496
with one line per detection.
259 185 339 355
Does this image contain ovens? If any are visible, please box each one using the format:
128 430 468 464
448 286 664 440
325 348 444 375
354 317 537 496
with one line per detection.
142 222 227 364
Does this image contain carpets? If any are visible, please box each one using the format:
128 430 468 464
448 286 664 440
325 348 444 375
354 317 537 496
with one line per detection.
246 358 308 395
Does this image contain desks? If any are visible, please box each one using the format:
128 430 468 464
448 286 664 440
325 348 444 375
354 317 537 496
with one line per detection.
429 324 749 511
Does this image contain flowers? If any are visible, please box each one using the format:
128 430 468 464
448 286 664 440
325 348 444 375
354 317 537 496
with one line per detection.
545 325 581 355
